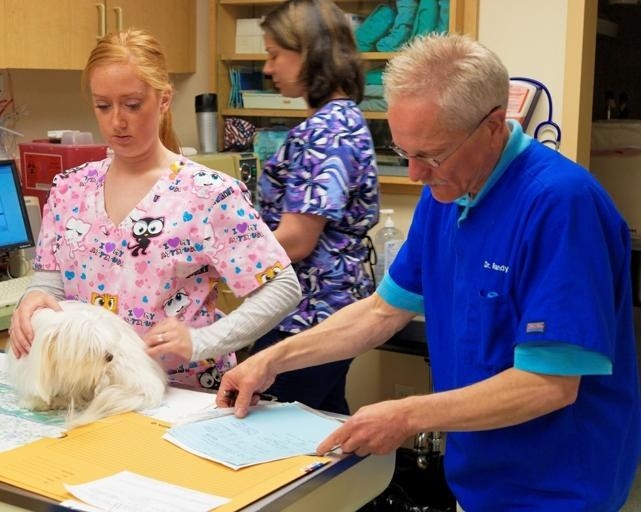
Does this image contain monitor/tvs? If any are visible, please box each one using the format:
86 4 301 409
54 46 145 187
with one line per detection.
0 159 36 250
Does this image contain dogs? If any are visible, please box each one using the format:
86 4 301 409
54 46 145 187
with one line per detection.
4 298 171 430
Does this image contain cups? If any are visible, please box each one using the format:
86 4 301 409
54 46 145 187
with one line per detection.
61 132 92 146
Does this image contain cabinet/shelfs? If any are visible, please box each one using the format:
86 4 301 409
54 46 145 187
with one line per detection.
0 0 195 74
209 0 478 194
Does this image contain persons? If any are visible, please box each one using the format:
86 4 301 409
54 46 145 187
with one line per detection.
9 28 303 390
216 30 641 512
254 0 380 415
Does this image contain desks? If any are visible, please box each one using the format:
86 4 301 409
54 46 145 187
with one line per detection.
0 382 396 512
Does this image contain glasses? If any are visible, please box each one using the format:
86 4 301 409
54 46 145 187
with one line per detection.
387 104 502 169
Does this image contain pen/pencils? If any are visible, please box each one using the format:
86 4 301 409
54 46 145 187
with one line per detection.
222 390 278 402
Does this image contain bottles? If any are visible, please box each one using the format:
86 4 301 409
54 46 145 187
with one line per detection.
604 90 629 120
195 93 217 153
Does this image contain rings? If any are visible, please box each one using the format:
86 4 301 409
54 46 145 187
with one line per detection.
157 334 164 342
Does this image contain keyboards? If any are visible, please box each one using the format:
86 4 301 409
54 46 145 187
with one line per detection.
0 275 32 307
